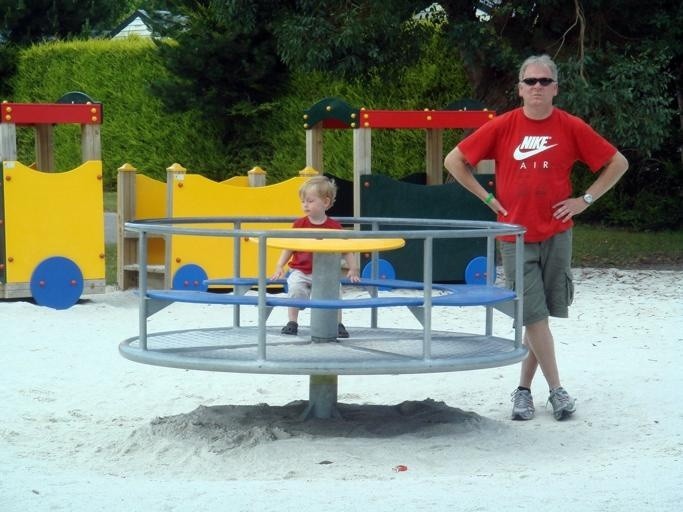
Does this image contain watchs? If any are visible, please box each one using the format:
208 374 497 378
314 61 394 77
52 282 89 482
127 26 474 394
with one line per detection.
582 193 595 205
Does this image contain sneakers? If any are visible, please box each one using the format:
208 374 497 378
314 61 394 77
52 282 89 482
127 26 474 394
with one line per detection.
511 388 534 420
548 387 576 421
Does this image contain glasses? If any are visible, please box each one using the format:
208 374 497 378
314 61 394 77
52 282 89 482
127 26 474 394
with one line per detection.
520 77 552 86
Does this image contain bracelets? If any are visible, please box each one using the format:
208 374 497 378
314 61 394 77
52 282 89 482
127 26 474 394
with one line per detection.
482 192 495 204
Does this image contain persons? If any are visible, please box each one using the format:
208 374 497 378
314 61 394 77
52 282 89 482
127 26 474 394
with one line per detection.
265 175 362 339
442 55 630 421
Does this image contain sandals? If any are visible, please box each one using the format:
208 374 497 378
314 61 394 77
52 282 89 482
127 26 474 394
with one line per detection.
281 321 298 335
337 323 348 337
